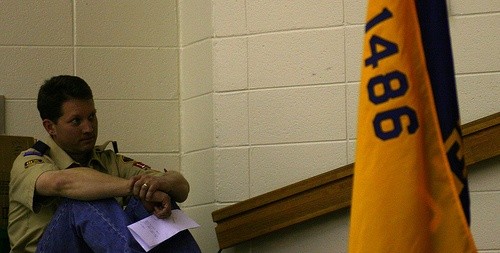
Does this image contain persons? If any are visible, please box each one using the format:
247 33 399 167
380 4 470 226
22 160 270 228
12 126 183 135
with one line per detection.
8 75 202 253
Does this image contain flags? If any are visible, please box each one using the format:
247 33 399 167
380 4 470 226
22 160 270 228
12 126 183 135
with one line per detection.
348 0 478 253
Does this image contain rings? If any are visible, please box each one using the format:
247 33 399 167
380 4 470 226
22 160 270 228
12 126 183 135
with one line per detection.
143 184 147 187
